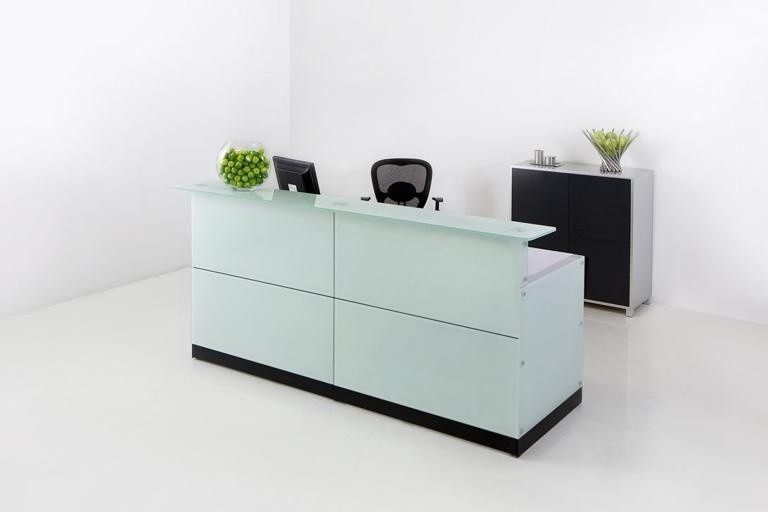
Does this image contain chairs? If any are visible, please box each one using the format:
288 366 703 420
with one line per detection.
361 157 444 210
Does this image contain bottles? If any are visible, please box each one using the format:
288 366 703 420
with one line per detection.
215 139 273 191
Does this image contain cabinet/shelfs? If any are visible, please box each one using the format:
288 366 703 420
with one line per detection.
511 160 653 317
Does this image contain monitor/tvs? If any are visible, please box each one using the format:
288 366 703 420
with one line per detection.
273 156 320 195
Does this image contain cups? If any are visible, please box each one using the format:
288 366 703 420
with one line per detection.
535 150 545 164
544 156 556 165
